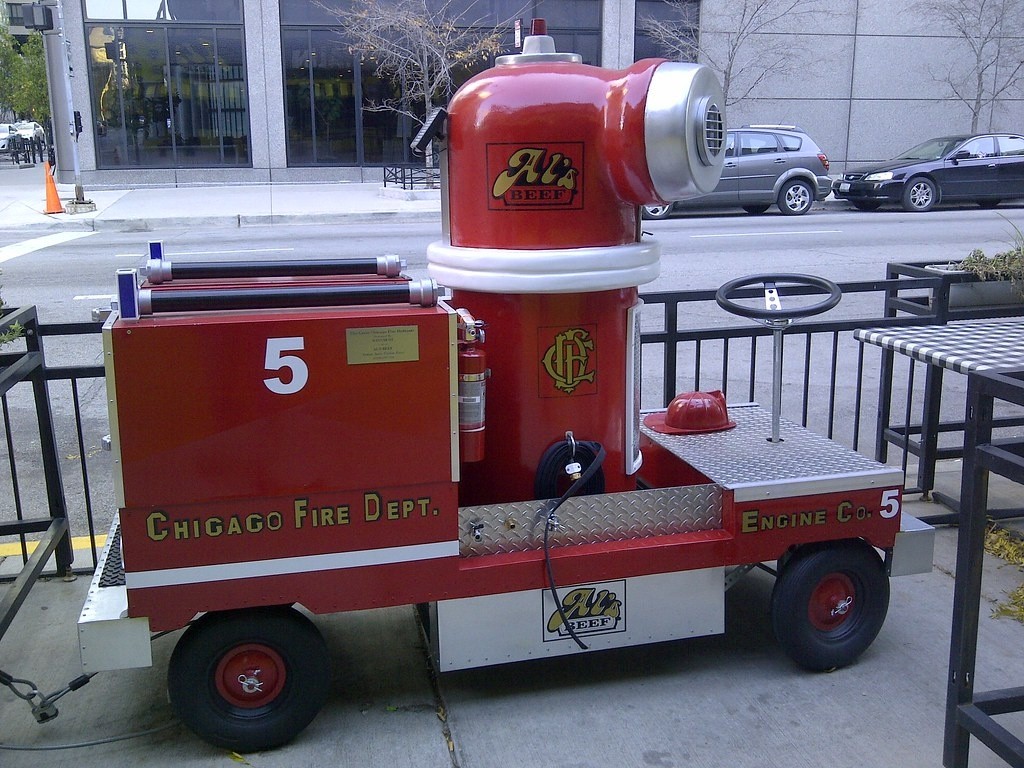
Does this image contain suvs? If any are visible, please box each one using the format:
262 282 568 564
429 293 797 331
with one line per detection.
641 123 834 222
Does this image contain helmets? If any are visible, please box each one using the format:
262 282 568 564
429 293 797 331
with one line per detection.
644 390 737 434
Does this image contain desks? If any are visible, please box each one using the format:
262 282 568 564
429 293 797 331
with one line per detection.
852 315 1024 542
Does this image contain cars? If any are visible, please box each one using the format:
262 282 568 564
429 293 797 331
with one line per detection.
831 131 1024 212
13 120 46 145
0 123 22 153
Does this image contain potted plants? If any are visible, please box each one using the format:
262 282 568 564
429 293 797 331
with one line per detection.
925 211 1024 307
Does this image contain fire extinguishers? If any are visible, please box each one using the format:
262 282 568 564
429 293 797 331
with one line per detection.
457 308 489 466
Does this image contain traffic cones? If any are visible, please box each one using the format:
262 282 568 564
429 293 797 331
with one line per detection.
43 160 67 214
